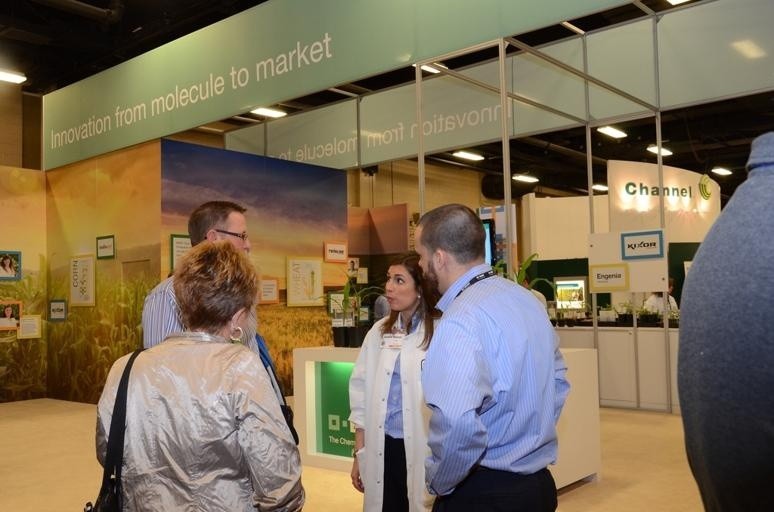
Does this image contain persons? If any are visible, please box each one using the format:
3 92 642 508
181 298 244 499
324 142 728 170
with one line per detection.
373 294 391 323
643 278 679 316
677 132 774 512
141 200 260 361
95 240 305 512
0 304 17 328
0 255 16 277
351 250 440 512
414 204 571 512
518 269 548 314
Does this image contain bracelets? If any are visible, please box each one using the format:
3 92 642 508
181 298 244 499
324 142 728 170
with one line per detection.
353 449 361 458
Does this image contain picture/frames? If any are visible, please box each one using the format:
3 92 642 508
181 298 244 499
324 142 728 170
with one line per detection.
0 235 117 336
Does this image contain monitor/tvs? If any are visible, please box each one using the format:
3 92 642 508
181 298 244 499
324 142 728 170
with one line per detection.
479 218 497 268
551 276 590 310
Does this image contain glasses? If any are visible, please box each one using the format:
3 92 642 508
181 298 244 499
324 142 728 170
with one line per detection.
204 229 248 241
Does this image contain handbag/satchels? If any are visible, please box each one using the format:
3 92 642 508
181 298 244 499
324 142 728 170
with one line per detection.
278 404 300 447
83 472 123 512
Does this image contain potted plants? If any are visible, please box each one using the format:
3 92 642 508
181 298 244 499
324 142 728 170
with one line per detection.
548 297 682 328
310 263 386 348
